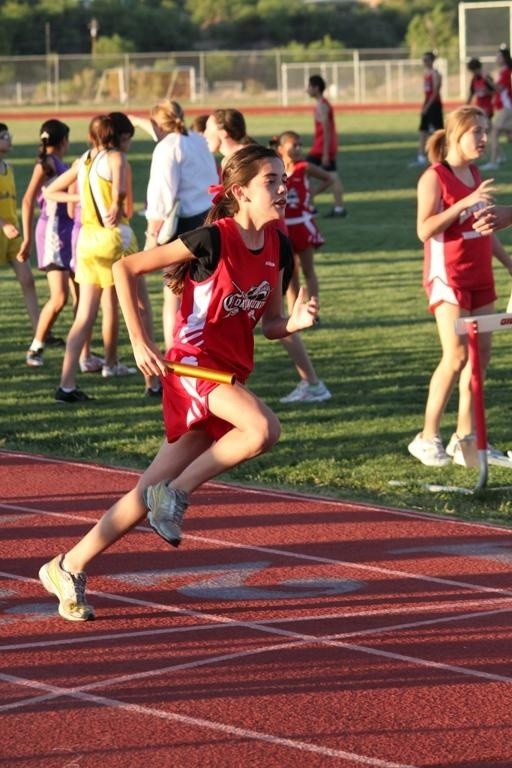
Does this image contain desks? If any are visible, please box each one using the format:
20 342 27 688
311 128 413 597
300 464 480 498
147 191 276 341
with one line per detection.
88 19 98 55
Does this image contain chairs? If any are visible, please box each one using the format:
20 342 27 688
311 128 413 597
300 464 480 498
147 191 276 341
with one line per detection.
405 429 452 469
143 383 164 404
139 478 190 549
79 354 105 372
477 161 498 170
446 431 504 466
311 317 321 330
25 346 45 368
321 206 348 220
409 156 426 167
44 333 64 349
101 359 139 379
36 552 97 625
54 386 97 403
276 378 333 404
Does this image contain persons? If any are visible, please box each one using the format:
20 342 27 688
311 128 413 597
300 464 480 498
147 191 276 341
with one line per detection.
303 74 346 218
478 48 512 170
16 120 104 372
273 129 335 316
1 122 64 346
142 102 218 351
189 114 210 132
203 108 333 401
407 49 444 166
35 141 320 622
471 202 512 235
405 103 512 468
466 57 508 165
44 112 163 402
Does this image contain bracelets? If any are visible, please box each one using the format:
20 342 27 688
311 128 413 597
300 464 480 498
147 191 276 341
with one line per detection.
146 229 158 238
